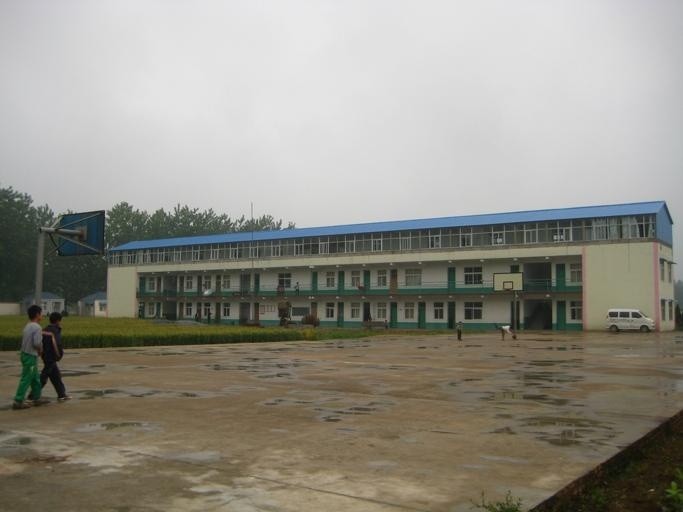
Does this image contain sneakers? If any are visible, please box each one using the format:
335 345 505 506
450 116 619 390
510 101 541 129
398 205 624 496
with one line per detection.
11 398 51 410
57 395 72 401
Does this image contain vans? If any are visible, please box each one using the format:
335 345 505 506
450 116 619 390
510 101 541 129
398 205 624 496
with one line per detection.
606 308 656 333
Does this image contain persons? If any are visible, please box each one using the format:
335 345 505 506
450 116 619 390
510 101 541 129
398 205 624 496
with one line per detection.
500 325 514 341
27 311 74 404
293 281 299 296
10 303 50 408
454 320 465 342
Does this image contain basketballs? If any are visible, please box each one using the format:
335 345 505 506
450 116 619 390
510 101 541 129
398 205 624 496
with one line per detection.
512 334 517 339
